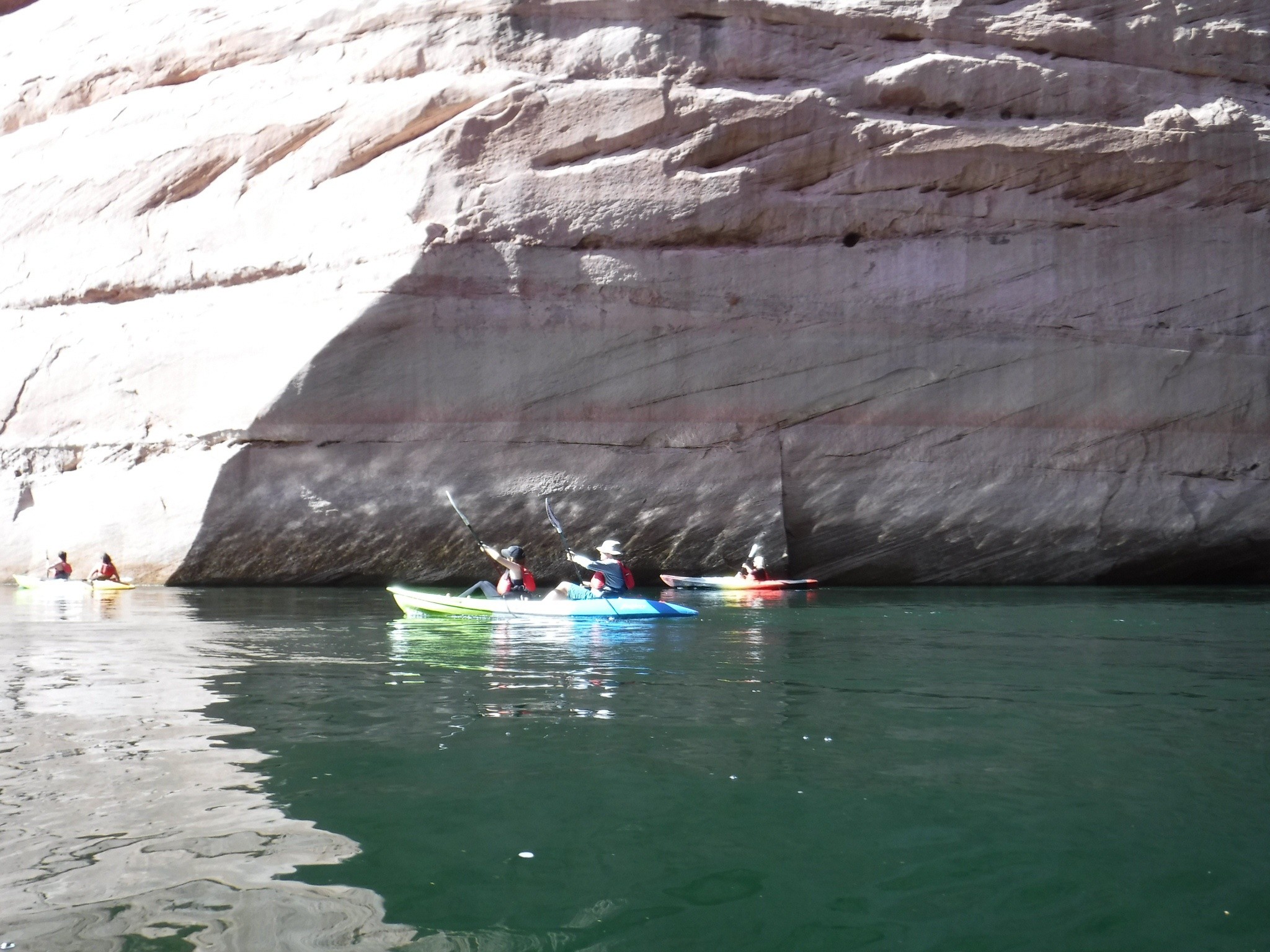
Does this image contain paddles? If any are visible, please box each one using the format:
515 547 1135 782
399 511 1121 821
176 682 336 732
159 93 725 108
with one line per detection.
39 576 135 586
737 544 758 575
545 498 583 586
446 489 501 577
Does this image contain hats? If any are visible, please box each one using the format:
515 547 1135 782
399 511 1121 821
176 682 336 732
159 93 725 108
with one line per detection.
500 545 526 560
595 539 624 557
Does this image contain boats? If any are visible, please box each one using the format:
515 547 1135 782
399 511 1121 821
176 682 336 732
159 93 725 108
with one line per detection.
386 585 700 619
660 572 820 590
12 573 136 591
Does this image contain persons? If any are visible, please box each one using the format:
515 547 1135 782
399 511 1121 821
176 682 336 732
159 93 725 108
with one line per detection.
446 544 536 601
46 551 73 579
520 539 635 601
734 556 769 581
87 552 120 582
